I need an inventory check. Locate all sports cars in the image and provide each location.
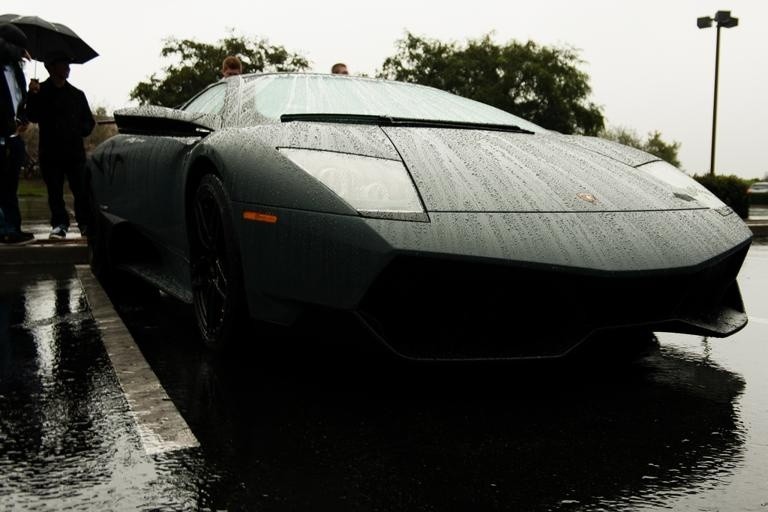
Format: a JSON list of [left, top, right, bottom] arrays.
[[86, 72, 754, 362]]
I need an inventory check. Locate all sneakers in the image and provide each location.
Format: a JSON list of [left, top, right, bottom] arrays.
[[49, 225, 67, 241], [0, 231, 34, 246]]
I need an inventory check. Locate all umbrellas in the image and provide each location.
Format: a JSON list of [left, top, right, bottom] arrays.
[[0, 16, 98, 93]]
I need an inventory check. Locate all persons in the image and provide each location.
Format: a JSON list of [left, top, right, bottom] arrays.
[[331, 63, 348, 74], [221, 55, 242, 80], [18, 54, 96, 240], [0, 23, 35, 243]]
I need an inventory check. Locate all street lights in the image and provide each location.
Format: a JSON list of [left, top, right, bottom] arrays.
[[697, 11, 738, 176]]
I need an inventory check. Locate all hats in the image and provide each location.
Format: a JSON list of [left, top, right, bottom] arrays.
[[1, 23, 32, 61]]
